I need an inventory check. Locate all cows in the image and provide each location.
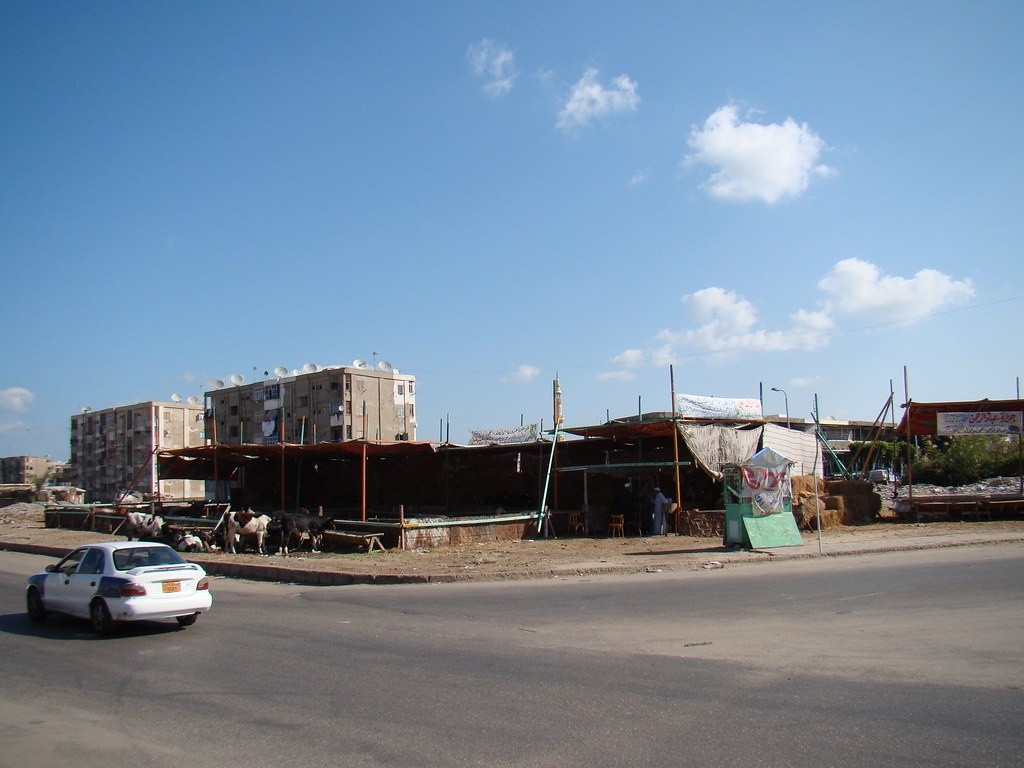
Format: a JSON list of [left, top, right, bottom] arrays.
[[170, 507, 336, 558], [126, 511, 170, 541]]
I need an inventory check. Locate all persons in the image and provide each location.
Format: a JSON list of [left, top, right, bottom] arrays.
[[649, 487, 673, 535]]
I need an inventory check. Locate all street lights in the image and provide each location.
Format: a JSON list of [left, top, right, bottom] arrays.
[[771, 387, 790, 429]]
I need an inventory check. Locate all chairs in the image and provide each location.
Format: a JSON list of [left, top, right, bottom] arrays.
[[147, 551, 163, 565], [566, 509, 585, 538], [85, 551, 98, 568], [607, 513, 625, 539]]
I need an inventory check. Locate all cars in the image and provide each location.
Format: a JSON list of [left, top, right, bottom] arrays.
[[25, 541, 214, 637]]
